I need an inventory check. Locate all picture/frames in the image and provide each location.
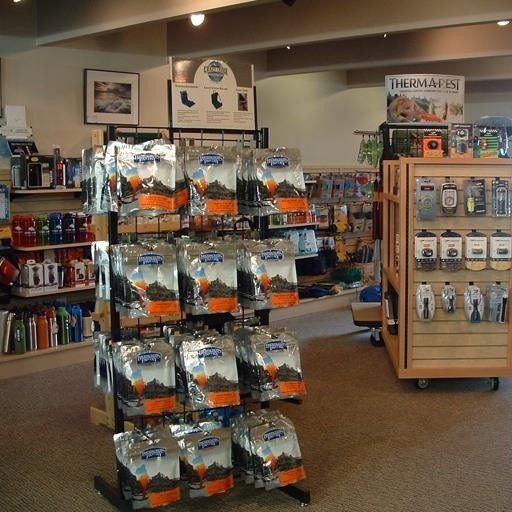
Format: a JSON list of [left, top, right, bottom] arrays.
[[82, 68, 142, 129]]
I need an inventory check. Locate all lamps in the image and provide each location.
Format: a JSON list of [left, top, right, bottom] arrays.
[[189, 13, 208, 28]]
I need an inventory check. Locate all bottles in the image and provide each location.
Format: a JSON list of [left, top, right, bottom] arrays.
[[11, 212, 95, 246], [1, 302, 92, 354]]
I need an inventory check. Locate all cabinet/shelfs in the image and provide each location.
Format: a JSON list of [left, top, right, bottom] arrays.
[[1, 184, 95, 365], [375, 154, 512, 394]]
